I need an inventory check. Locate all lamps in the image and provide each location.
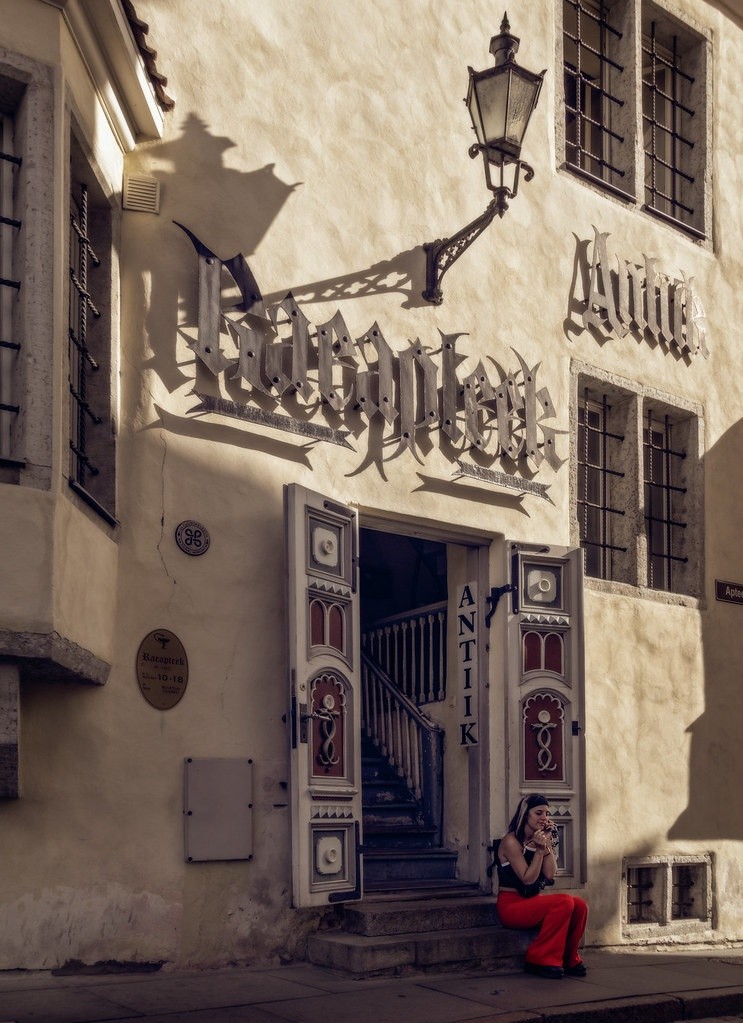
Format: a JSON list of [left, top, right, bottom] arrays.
[[419, 11, 550, 308]]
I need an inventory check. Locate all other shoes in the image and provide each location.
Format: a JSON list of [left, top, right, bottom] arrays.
[[565, 965, 587, 977], [524, 961, 565, 979]]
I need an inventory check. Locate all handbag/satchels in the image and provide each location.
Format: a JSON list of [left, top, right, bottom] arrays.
[[516, 883, 539, 897]]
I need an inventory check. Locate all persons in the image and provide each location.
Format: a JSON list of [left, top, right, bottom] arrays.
[[492, 793, 589, 978]]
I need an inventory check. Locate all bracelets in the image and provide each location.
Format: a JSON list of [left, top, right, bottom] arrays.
[[544, 852, 551, 858]]
